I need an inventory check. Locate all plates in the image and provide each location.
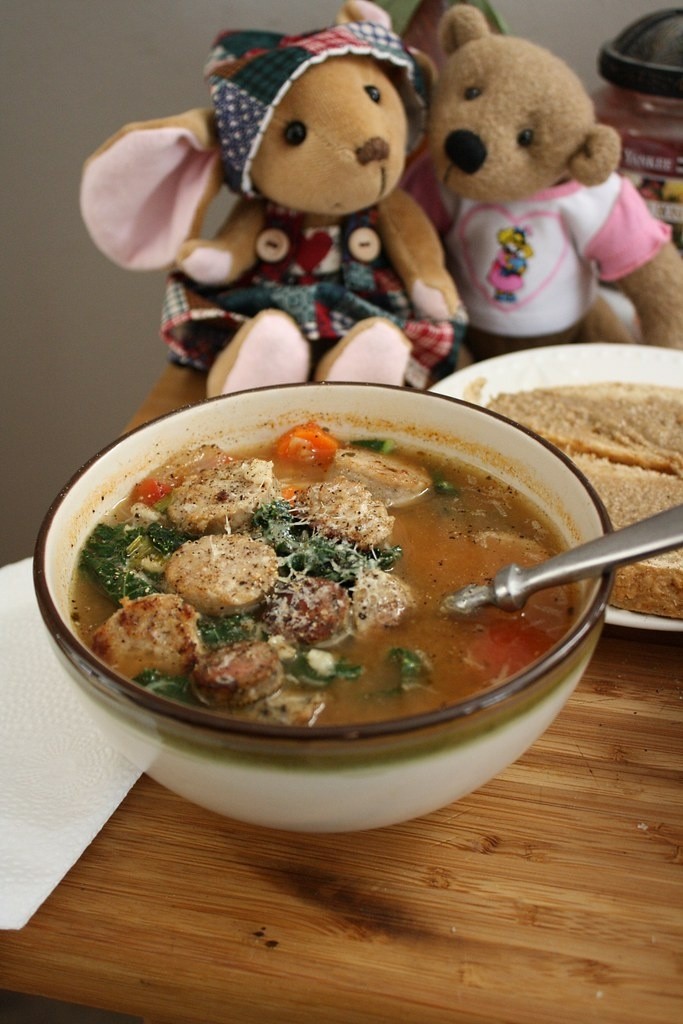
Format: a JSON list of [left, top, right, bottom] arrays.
[[422, 339, 683, 636]]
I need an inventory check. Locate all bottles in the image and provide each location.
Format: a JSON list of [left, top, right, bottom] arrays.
[[580, 8, 683, 255]]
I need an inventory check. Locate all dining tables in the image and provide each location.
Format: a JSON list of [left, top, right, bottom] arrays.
[[0, 359, 683, 1024]]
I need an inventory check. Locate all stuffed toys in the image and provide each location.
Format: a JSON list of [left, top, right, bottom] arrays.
[[393, 0, 681, 359], [73, 3, 468, 392]]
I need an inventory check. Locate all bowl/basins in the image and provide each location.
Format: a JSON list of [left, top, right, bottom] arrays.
[[30, 380, 618, 830]]
